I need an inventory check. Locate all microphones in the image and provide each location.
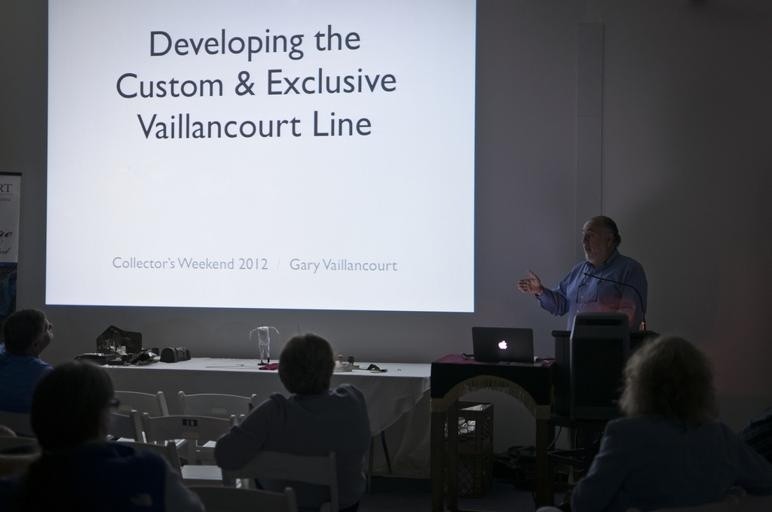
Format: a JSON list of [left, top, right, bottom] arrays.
[[583, 272, 647, 332]]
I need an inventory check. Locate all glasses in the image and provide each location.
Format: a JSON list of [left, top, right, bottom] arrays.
[[95, 397, 122, 415]]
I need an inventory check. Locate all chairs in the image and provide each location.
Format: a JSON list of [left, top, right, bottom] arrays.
[[142, 411, 250, 490], [163, 469, 297, 511], [106, 409, 143, 444], [2, 411, 44, 509], [112, 391, 193, 463], [178, 391, 258, 461]]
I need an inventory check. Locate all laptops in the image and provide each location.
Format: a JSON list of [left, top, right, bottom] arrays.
[[472, 327, 537, 362]]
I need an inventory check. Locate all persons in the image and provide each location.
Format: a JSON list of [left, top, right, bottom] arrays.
[[2, 309, 54, 411], [517, 216, 648, 332], [2, 359, 205, 511], [570, 336, 772, 512], [215, 334, 371, 511]]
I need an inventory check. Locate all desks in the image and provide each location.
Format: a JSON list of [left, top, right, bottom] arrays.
[[80, 356, 430, 481]]
